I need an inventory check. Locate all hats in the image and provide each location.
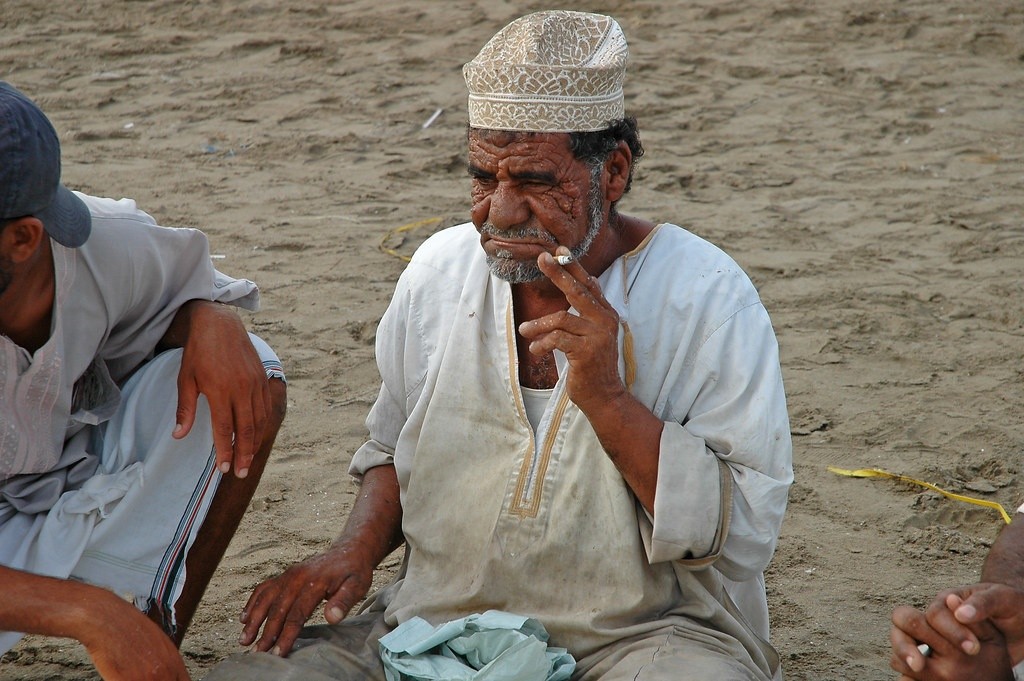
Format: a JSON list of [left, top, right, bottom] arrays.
[[0, 80, 91, 248], [462, 10, 629, 132]]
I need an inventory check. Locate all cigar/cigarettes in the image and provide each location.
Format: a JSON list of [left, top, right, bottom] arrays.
[[553, 256, 572, 265]]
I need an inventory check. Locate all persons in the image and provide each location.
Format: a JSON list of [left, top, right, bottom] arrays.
[[890, 503, 1024, 681], [206, 10, 794, 681], [0, 81, 288, 681]]
